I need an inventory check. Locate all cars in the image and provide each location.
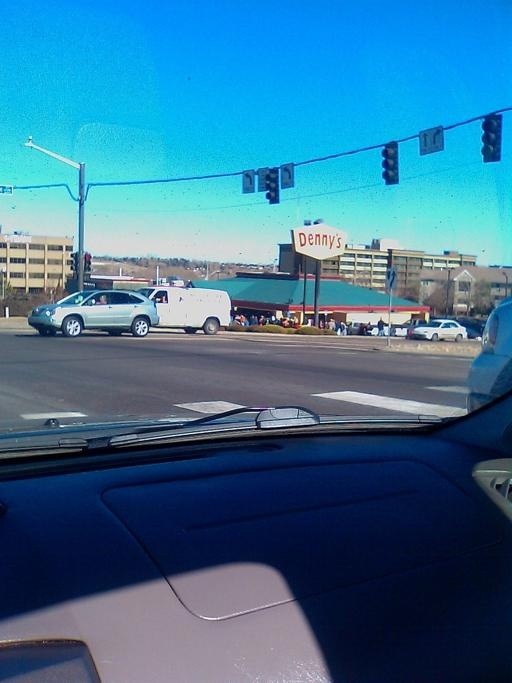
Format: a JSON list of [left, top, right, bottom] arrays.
[[409, 318, 468, 343], [347, 321, 366, 335], [370, 323, 409, 336]]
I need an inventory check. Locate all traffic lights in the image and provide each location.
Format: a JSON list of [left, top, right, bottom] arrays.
[[381, 141, 400, 186], [480, 113, 503, 163], [84, 251, 91, 272], [70, 251, 78, 271], [264, 167, 281, 205]]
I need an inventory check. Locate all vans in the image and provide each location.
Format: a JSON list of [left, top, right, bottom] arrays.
[[134, 286, 232, 335]]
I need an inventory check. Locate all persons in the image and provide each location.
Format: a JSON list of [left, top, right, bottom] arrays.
[[377, 316, 386, 336], [158, 293, 167, 304], [95, 294, 107, 305], [233, 313, 349, 335]]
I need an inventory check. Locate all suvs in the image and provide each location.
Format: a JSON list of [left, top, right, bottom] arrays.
[[26, 288, 161, 338]]
